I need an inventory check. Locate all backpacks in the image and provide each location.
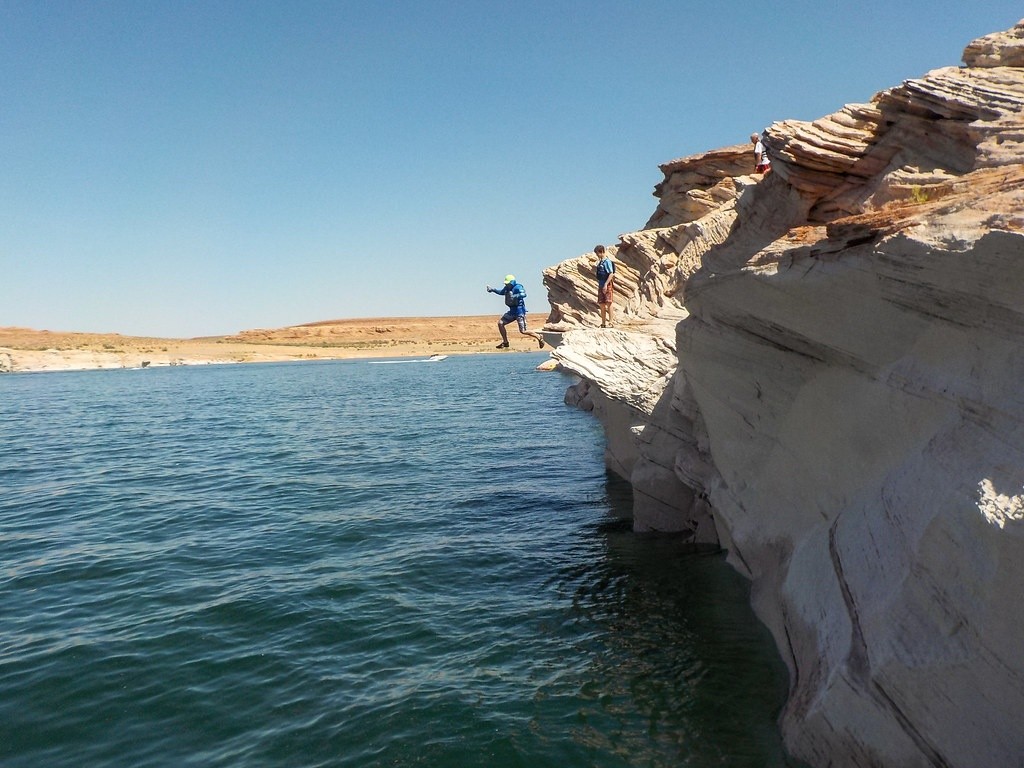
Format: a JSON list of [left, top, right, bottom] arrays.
[[599, 258, 616, 274]]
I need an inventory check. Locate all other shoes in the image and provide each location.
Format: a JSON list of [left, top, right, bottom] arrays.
[[608, 323, 614, 328], [598, 324, 605, 328], [496, 342, 509, 348], [539, 334, 544, 348]]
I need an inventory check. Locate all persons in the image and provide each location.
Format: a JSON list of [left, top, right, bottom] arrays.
[[487, 275, 545, 349], [594, 245, 614, 328]]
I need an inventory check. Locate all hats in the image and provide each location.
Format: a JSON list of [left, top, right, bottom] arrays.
[[503, 275, 516, 286]]
